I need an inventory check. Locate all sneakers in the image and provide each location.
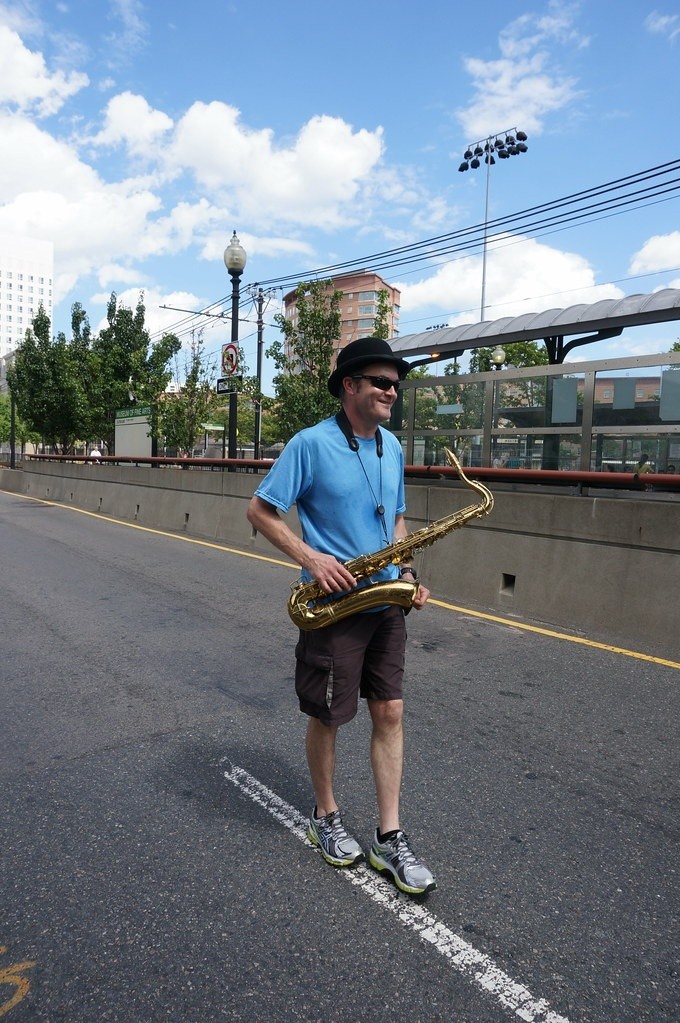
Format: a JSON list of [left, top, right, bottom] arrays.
[[369, 828, 438, 896], [306, 805, 366, 868]]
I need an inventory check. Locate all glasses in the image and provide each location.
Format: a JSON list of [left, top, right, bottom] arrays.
[[351, 375, 400, 393]]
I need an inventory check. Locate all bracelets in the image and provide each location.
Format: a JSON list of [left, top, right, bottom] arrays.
[[401, 568, 416, 580]]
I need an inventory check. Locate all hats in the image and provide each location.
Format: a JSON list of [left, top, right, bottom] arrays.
[[327, 337, 411, 399]]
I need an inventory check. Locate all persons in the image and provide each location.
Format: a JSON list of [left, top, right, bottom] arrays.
[[625, 454, 675, 474], [246, 337, 439, 894], [492, 449, 522, 469], [90, 447, 101, 465], [181, 452, 190, 470]]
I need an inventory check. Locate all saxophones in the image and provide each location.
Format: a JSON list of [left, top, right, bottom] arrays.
[[286, 446, 496, 631]]
